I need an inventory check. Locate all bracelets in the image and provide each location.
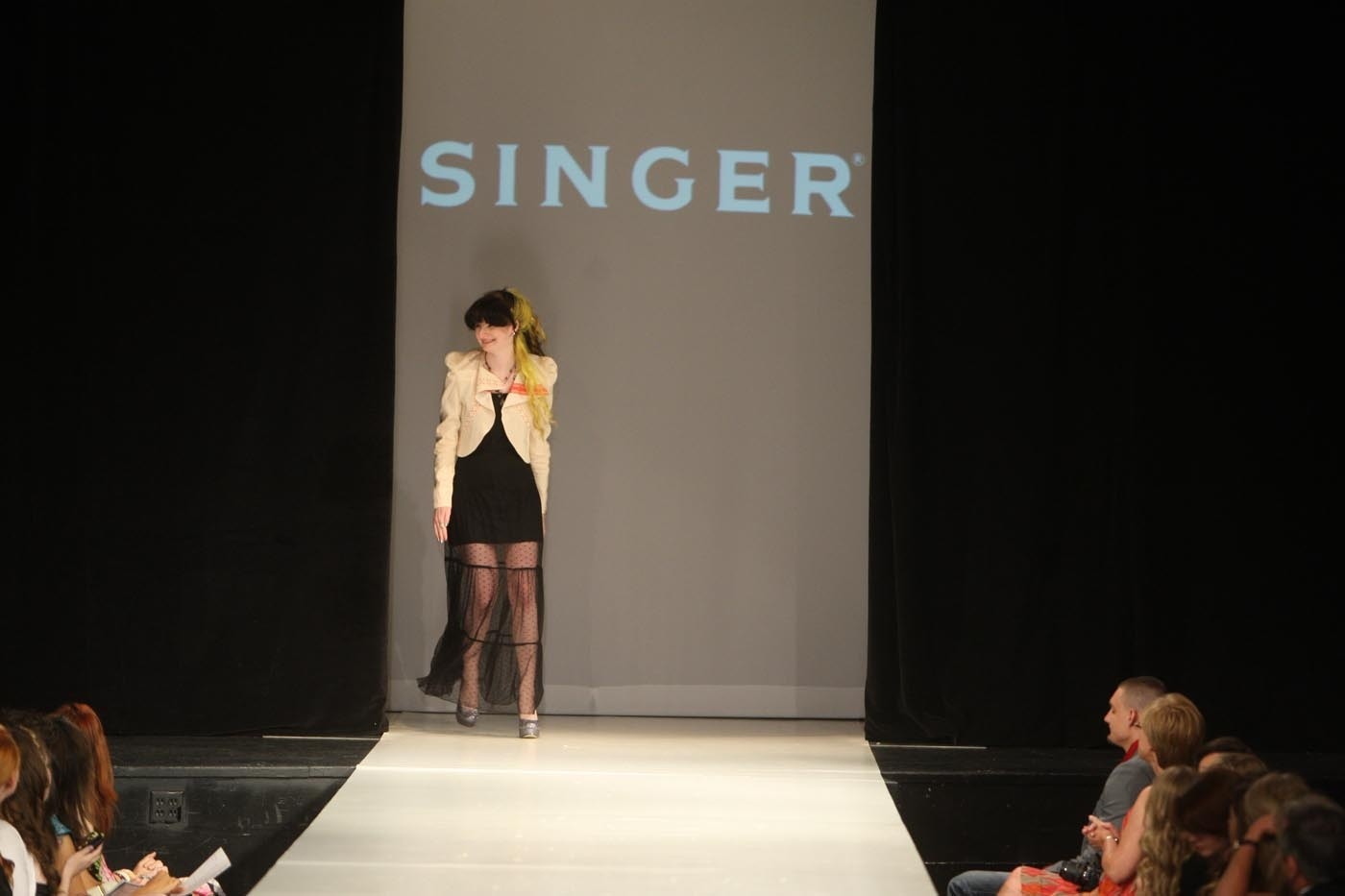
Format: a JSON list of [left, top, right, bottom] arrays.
[[1102, 834, 1121, 844]]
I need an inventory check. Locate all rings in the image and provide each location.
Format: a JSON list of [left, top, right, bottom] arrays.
[[439, 522, 446, 530]]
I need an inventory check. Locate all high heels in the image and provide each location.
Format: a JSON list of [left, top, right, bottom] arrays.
[[518, 712, 540, 740], [456, 698, 479, 727]]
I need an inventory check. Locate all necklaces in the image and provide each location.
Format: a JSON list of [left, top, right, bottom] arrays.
[[484, 350, 519, 399]]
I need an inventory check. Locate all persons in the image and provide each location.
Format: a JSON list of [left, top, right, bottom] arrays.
[[946, 675, 1161, 895], [1132, 730, 1344, 896], [0, 696, 231, 895], [416, 288, 558, 739], [996, 690, 1209, 896]]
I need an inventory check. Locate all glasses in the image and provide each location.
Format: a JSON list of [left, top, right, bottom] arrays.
[[1134, 722, 1144, 732]]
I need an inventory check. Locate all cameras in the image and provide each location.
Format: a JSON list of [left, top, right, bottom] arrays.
[[1058, 858, 1100, 890]]
